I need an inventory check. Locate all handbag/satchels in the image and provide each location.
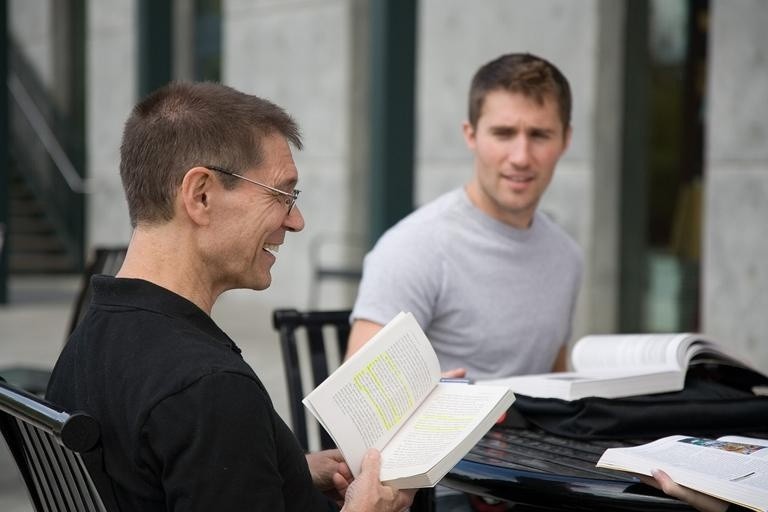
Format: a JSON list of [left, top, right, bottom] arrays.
[[503, 373, 768, 445]]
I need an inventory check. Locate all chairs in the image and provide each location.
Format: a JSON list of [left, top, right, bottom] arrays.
[[270, 306, 359, 460], [0, 374, 119, 509]]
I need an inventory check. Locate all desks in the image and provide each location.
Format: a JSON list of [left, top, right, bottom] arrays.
[[410, 427, 703, 509]]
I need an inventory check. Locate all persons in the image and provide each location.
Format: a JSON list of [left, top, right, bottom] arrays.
[[343, 52, 573, 376], [46, 77, 418, 511], [622, 467, 768, 512]]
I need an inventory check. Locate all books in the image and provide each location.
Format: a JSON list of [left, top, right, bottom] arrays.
[[303, 312, 515, 489], [488, 331, 768, 403], [595, 435, 768, 511]]
